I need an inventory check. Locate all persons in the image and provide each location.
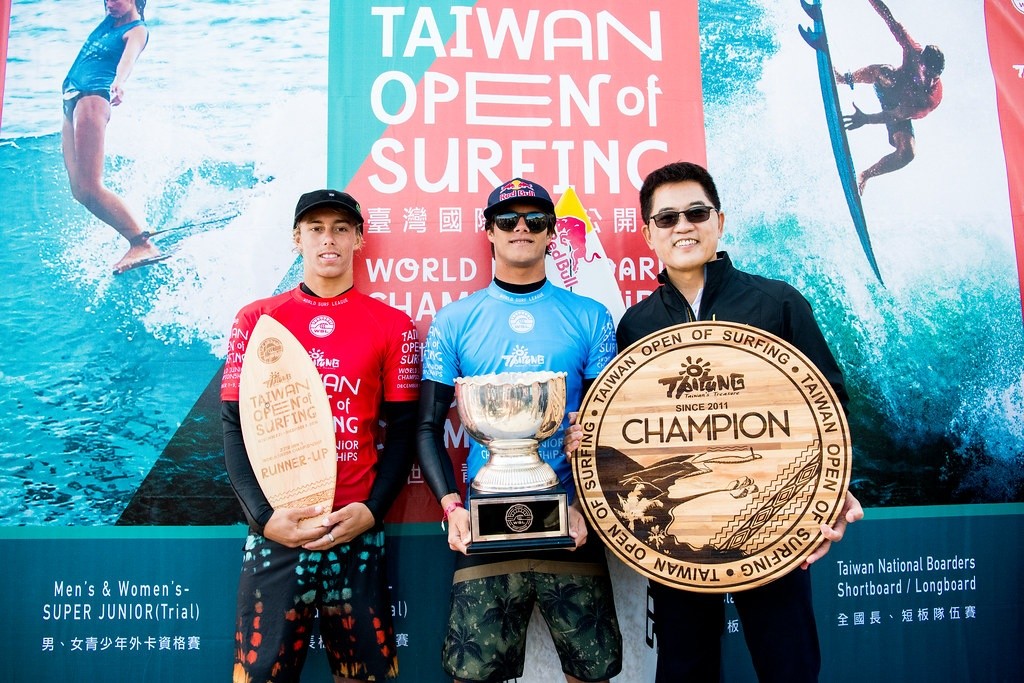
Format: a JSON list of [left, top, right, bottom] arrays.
[[832, 0, 946, 196], [61, 0, 163, 273], [416, 175, 622, 683], [618, 161, 863, 683], [219, 189, 421, 683]]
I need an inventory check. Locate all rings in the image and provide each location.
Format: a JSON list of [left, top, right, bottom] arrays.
[[328, 533, 334, 542]]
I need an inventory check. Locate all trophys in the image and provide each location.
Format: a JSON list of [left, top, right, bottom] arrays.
[[451, 368, 576, 554]]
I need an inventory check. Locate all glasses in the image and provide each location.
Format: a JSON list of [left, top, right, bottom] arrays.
[[647, 206, 718, 228], [489, 211, 555, 233]]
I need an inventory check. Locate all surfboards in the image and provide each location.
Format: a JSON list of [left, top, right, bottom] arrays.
[[111, 249, 178, 274], [795, 0, 887, 291], [235, 313, 338, 530]]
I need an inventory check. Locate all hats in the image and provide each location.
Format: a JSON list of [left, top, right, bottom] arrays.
[[483, 178, 556, 233], [294, 189, 364, 236]]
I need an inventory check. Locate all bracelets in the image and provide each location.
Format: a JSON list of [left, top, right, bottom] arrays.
[[868, 114, 871, 123], [440, 501, 465, 529]]
